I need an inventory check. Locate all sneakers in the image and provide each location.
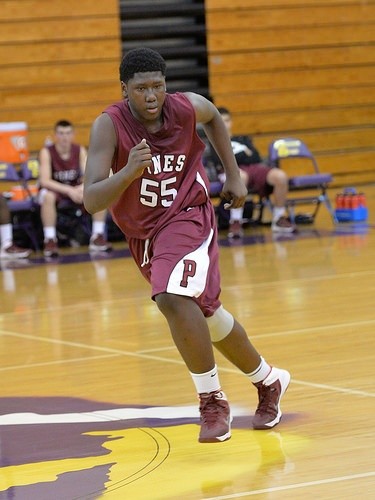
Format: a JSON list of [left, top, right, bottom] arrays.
[[198, 391, 233, 443], [251, 365, 291, 430], [89, 234, 111, 251], [43, 239, 59, 257], [228, 220, 243, 238], [271, 216, 296, 233], [0, 245, 33, 258]]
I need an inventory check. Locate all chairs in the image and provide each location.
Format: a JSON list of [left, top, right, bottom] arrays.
[[8, 139, 338, 252]]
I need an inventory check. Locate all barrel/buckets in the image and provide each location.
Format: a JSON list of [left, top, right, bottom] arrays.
[[0, 122, 28, 164]]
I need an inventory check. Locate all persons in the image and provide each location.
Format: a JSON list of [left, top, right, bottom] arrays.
[[215, 107, 298, 238], [83, 50, 291, 443], [36, 120, 114, 255], [0, 195, 34, 258]]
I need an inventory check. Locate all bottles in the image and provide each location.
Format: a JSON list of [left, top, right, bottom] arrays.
[[336, 191, 367, 210]]
